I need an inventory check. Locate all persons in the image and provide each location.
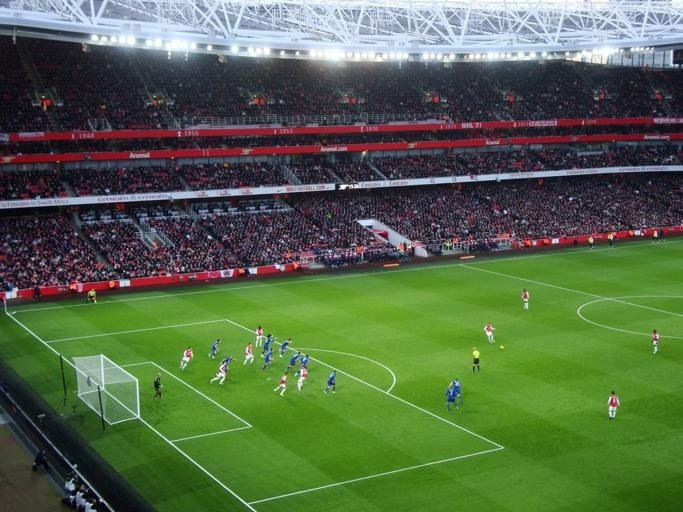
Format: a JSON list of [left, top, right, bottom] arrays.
[[441, 382, 460, 414], [606, 390, 620, 421], [208, 338, 223, 360], [521, 287, 529, 309], [322, 370, 336, 395], [255, 324, 311, 397], [63, 474, 100, 512], [1, 32, 683, 302], [450, 378, 462, 405], [210, 362, 227, 385], [483, 321, 495, 344], [471, 346, 480, 375], [650, 328, 659, 355], [243, 341, 254, 367], [34, 448, 52, 473], [151, 370, 164, 402], [219, 355, 232, 371], [178, 345, 193, 372]]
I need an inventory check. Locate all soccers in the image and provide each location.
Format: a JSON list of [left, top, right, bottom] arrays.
[[500, 346, 504, 350]]
